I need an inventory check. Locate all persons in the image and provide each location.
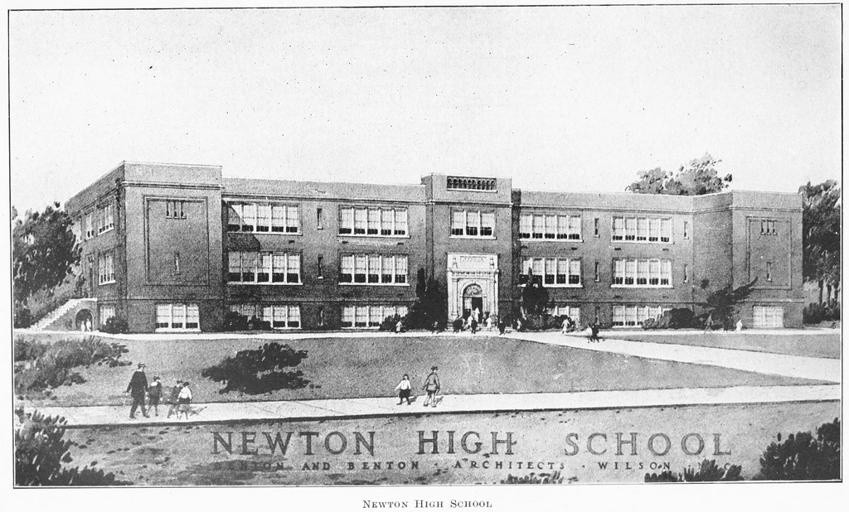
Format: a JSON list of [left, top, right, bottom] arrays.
[[125, 364, 192, 419], [423, 367, 441, 408], [394, 373, 412, 405], [393, 305, 599, 343], [76, 314, 92, 332], [701, 313, 743, 332]]
